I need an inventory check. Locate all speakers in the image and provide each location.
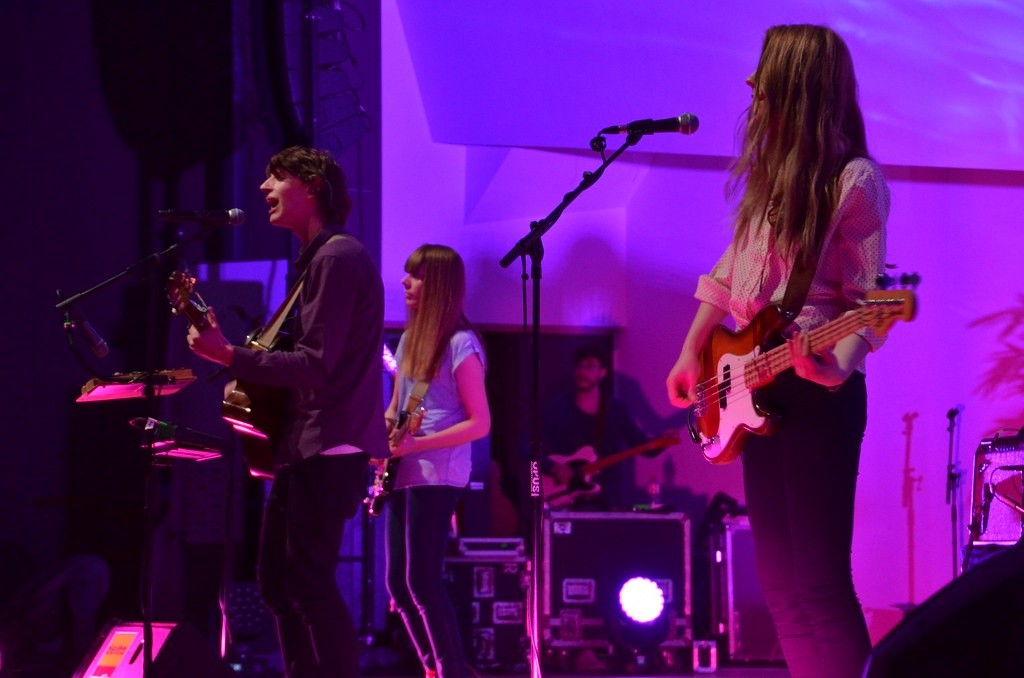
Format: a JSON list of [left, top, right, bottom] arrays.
[[862, 436, 1024, 678], [70, 619, 240, 678], [719, 515, 786, 662]]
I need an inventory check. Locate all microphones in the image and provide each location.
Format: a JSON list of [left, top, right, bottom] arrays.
[[602, 113, 698, 135], [65, 303, 109, 359], [158, 209, 246, 226], [949, 407, 963, 418], [999, 465, 1024, 472]]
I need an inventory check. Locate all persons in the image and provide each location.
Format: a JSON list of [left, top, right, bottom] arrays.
[[666, 23, 892, 678], [186, 145, 492, 678], [535, 341, 680, 674]]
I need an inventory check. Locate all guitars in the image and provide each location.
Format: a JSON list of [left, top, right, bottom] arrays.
[[362, 379, 429, 519], [165, 269, 299, 480], [541, 429, 683, 509], [692, 269, 922, 463]]
